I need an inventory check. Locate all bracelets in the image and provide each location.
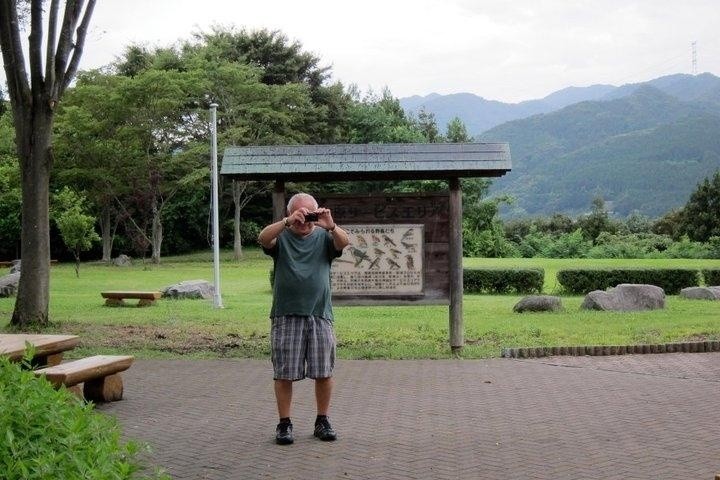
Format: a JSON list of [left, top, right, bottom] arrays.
[[326, 223, 336, 233], [283, 218, 294, 229]]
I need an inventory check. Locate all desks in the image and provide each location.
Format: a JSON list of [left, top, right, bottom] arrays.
[[0, 333, 80, 372]]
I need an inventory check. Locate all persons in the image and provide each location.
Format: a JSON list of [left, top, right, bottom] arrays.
[[256, 192, 350, 446]]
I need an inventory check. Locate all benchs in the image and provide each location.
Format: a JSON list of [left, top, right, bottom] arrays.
[[31, 354, 135, 405], [101, 291, 162, 308]]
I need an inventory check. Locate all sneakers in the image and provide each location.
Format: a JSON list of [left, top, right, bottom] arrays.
[[314, 418, 336, 441], [275, 419, 294, 444]]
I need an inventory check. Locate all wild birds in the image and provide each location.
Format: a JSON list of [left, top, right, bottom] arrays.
[[401, 229, 415, 241], [401, 242, 417, 250], [382, 235, 397, 247], [389, 249, 402, 254], [373, 248, 385, 254], [347, 247, 369, 260], [369, 257, 380, 270], [386, 257, 401, 268], [356, 236, 367, 247], [372, 235, 380, 242], [404, 255, 414, 268]]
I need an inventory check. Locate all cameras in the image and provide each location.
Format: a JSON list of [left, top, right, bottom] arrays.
[[303, 213, 320, 224]]
